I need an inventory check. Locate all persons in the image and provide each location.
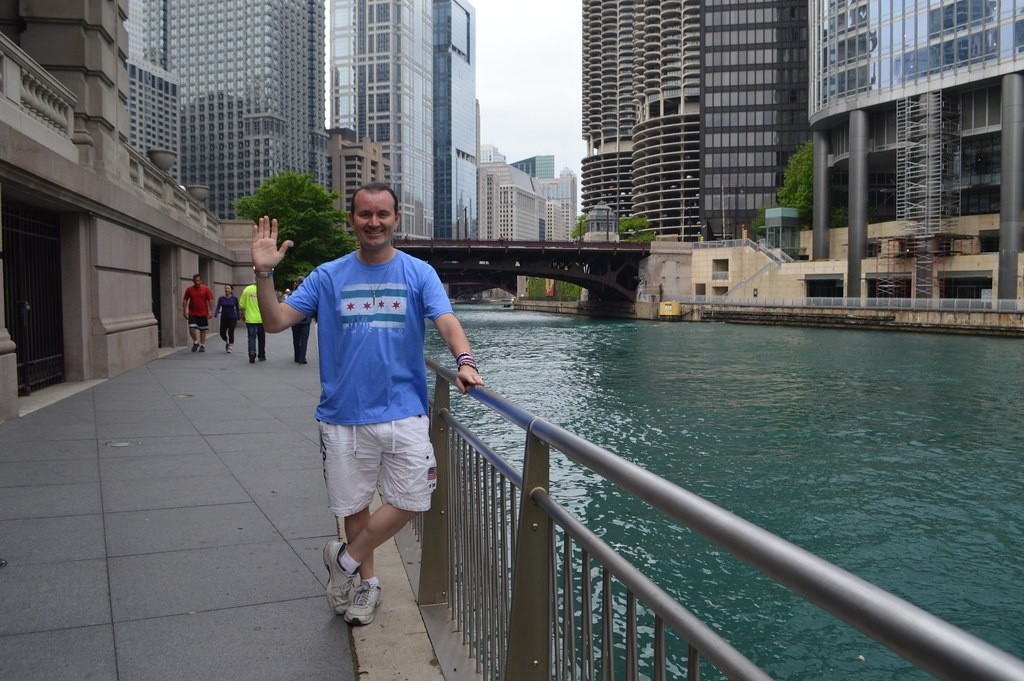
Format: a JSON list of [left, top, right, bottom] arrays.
[[282, 288, 291, 300], [251, 182, 487, 627], [239, 279, 268, 363], [213, 283, 241, 353], [183, 273, 214, 352], [288, 277, 318, 365]]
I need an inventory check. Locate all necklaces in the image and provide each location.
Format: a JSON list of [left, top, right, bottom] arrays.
[[359, 249, 394, 305]]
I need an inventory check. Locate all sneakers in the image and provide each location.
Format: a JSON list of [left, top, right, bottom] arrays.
[[323, 541, 357, 615], [226, 344, 229, 350], [227, 347, 232, 352], [344, 580, 382, 625]]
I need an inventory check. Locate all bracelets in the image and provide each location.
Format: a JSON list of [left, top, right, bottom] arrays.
[[253, 266, 274, 278], [456, 352, 478, 370]]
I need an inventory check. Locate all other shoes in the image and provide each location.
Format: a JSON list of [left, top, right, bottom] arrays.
[[259, 356, 266, 361], [199, 346, 204, 352], [192, 343, 199, 351], [250, 352, 256, 363]]
